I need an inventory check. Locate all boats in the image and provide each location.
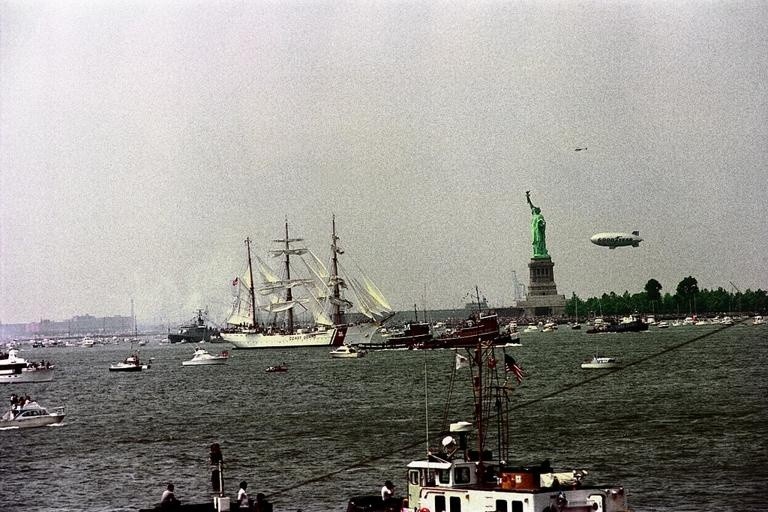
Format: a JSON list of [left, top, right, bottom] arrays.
[[109, 343, 152, 372], [0, 305, 223, 350], [0, 350, 55, 383], [346, 326, 627, 512], [265, 361, 289, 372], [356, 285, 500, 351], [0, 400, 66, 429], [453, 319, 520, 349], [181, 339, 228, 366], [328, 339, 368, 359], [580, 345, 626, 369], [504, 295, 766, 333]]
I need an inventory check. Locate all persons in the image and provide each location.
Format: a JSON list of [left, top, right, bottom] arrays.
[[381, 480, 396, 511], [236, 480, 250, 509], [9, 392, 32, 411], [26, 359, 51, 371], [161, 483, 180, 509], [254, 493, 272, 511], [525, 191, 549, 257]]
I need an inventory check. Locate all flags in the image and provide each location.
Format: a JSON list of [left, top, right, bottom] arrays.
[[505, 355, 524, 384], [454, 353, 467, 371]]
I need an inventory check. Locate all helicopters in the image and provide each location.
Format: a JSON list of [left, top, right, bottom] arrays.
[[572, 145, 588, 152]]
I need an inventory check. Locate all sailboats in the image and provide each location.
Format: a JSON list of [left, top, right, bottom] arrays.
[[220, 212, 396, 350]]
